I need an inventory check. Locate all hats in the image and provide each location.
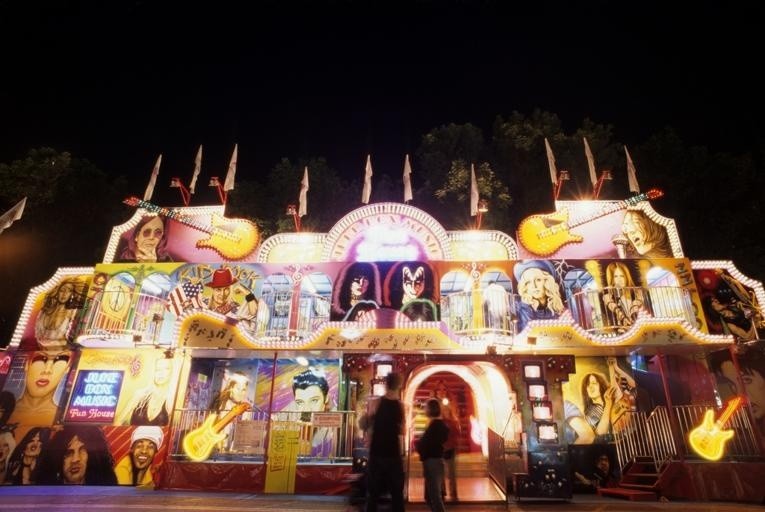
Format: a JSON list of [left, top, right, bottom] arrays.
[[129, 425, 163, 451], [513, 259, 556, 280], [205, 270, 236, 289]]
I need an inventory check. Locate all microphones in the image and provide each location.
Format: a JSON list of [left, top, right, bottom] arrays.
[[612, 234, 629, 259]]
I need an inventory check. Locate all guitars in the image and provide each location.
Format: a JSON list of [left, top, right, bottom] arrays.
[[518, 188, 663, 257], [689, 398, 742, 461], [123, 196, 261, 261], [182, 403, 252, 462]]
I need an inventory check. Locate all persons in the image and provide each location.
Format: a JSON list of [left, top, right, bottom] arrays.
[[562, 400, 596, 447], [602, 260, 647, 334], [417, 398, 448, 511], [571, 452, 613, 495], [290, 370, 338, 461], [358, 371, 408, 512], [386, 262, 435, 326], [514, 267, 566, 334], [440, 401, 462, 498], [622, 207, 673, 259], [332, 260, 383, 322], [481, 279, 511, 331], [584, 355, 639, 438]]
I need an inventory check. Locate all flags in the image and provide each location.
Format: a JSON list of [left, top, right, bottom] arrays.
[[403, 155, 414, 203], [470, 165, 480, 215], [0, 197, 26, 233], [224, 143, 239, 190], [623, 146, 640, 194], [545, 139, 557, 184], [298, 165, 310, 217], [584, 139, 598, 187], [143, 155, 163, 203], [189, 144, 202, 196], [361, 154, 373, 205]]
